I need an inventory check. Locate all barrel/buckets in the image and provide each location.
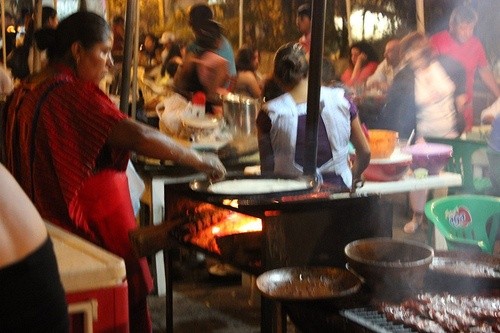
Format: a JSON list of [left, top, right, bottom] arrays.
[[218, 93, 263, 144]]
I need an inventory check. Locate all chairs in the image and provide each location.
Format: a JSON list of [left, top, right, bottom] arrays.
[[424, 135, 493, 195], [425, 194, 500, 255]]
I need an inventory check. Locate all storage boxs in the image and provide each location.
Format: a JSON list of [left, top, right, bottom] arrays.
[[45, 222, 130, 333]]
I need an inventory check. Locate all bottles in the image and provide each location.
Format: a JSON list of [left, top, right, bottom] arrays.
[[185, 91, 207, 121]]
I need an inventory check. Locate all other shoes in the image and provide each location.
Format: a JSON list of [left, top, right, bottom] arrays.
[[404, 221, 423, 233]]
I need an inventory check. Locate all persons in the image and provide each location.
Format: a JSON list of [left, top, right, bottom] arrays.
[[0, 0, 500, 194], [0, 10, 227, 333], [0, 162, 69, 333]]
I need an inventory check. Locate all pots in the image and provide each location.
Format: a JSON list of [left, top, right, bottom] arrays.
[[186, 165, 323, 204]]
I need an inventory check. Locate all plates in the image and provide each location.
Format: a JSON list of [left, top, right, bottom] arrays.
[[429, 251, 500, 291], [257, 265, 361, 303]]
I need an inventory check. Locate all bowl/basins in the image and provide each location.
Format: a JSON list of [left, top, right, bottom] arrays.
[[344, 238, 436, 303], [350, 152, 413, 183], [363, 128, 399, 160], [405, 143, 454, 175]]
